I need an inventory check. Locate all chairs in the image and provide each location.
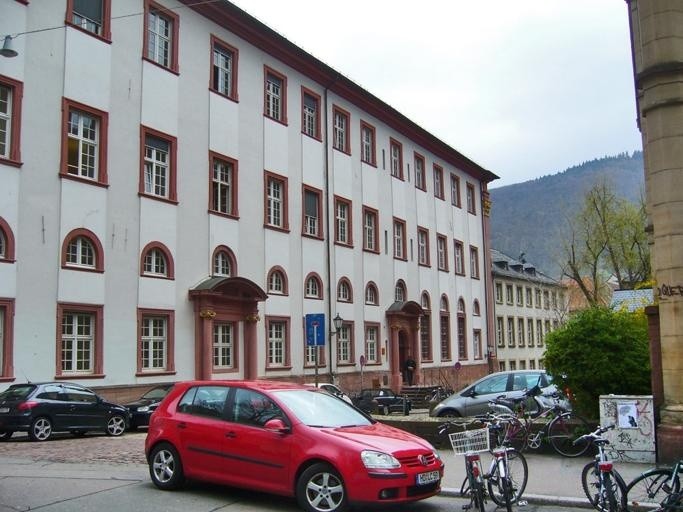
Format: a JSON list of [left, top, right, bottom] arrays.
[[236, 391, 256, 421]]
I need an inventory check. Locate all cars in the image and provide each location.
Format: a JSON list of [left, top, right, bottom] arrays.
[[431, 369, 577, 417], [303, 381, 355, 405], [143, 379, 445, 512], [0, 381, 131, 443], [122, 381, 221, 432], [351, 387, 412, 416]]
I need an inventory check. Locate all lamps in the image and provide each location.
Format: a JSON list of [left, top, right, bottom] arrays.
[[329, 312, 343, 336]]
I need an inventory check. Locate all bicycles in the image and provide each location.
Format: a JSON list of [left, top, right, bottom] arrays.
[[437, 376, 683, 512]]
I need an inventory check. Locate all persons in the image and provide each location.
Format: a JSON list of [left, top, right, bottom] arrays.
[[406, 356, 416, 386]]
